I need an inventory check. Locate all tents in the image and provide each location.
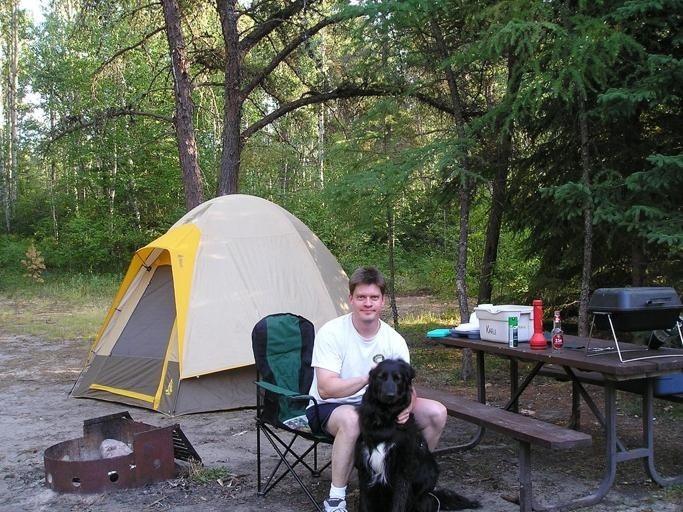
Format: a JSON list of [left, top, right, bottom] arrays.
[[68, 194, 352, 417]]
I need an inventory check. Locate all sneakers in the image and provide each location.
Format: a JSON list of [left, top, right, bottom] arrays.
[[323, 497, 348, 512]]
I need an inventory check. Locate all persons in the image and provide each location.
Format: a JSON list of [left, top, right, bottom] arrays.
[[305, 267, 447, 512]]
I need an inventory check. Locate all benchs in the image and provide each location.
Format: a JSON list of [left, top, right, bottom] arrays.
[[538, 364, 683, 432], [414, 384, 593, 512]]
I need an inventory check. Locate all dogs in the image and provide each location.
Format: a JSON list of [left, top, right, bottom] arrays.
[[354, 357, 484, 512]]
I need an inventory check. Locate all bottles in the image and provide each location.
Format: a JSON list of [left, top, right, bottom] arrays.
[[551, 311, 565, 351], [508, 316, 518, 349]]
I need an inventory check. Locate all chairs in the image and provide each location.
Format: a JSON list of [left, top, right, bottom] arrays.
[[252, 313, 333, 512]]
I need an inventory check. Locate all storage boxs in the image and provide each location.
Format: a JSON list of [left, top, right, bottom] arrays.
[[584, 287, 683, 363], [624, 372, 683, 400], [474, 305, 534, 344]]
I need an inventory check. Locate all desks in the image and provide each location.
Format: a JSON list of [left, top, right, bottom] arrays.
[[423, 333, 683, 512]]
